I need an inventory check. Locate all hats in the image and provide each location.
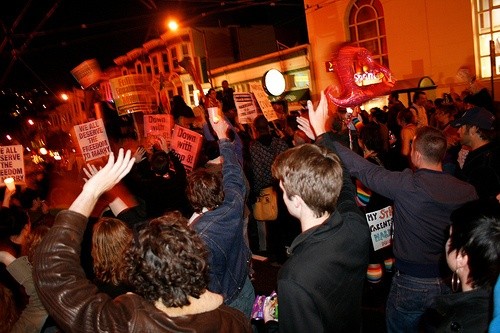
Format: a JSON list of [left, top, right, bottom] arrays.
[[463, 94, 483, 107], [450, 107, 495, 130]]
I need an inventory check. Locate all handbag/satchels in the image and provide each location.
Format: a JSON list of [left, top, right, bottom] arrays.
[[252, 186, 278, 220]]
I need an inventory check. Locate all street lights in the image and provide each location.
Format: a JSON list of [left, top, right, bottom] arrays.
[[167, 19, 213, 89]]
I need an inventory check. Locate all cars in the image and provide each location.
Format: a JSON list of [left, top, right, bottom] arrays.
[[0, 132, 46, 202], [42, 129, 77, 177]]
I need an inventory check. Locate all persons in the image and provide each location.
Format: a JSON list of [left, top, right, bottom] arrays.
[[271, 90, 370, 333], [0, 81, 500, 333]]
